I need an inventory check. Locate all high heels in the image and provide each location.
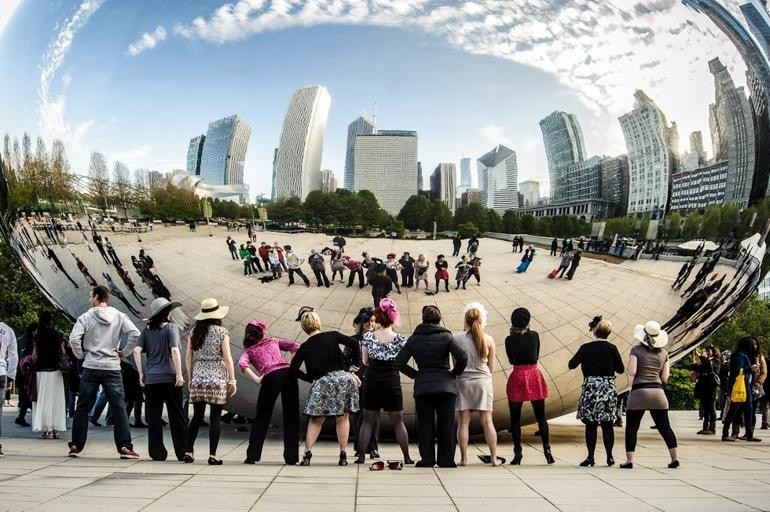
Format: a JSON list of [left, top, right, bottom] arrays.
[[607, 457, 614, 466], [579, 458, 595, 467], [544, 449, 555, 464], [39, 431, 53, 439], [53, 431, 60, 439], [338, 451, 348, 466], [510, 454, 523, 465], [300, 450, 312, 466]]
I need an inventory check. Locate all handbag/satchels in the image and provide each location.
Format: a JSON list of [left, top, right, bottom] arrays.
[[709, 357, 721, 388], [58, 353, 74, 374], [731, 368, 747, 403]]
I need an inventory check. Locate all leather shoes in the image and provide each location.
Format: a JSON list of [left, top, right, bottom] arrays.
[[15, 417, 30, 426], [386, 461, 404, 470], [184, 454, 194, 463], [370, 450, 380, 459], [620, 463, 632, 468], [369, 460, 389, 471], [88, 414, 209, 427], [477, 455, 506, 463], [209, 458, 222, 465], [697, 430, 761, 441], [668, 461, 679, 468]]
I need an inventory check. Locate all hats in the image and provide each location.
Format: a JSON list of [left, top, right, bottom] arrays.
[[310, 249, 321, 255], [142, 297, 183, 322], [354, 307, 374, 324], [333, 248, 342, 252], [379, 297, 399, 321], [197, 298, 230, 321], [511, 308, 530, 328], [248, 320, 268, 330], [589, 315, 602, 331], [463, 301, 488, 327], [634, 320, 669, 348]]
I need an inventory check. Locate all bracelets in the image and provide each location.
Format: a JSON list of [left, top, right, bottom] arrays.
[[228, 379, 237, 384], [188, 376, 191, 381]]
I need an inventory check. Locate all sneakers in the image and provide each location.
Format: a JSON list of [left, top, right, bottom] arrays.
[[120, 447, 140, 459], [69, 445, 80, 457]]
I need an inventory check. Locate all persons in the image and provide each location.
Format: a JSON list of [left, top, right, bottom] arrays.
[[237, 318, 302, 464], [392, 304, 469, 467], [355, 298, 414, 465], [0, 306, 248, 439], [618, 320, 680, 468], [225, 222, 482, 307], [132, 296, 191, 460], [504, 307, 555, 465], [687, 337, 770, 442], [343, 306, 383, 459], [452, 301, 504, 467], [640, 224, 760, 335], [565, 315, 625, 466], [290, 305, 363, 466], [550, 236, 627, 280], [11, 214, 197, 313], [512, 235, 535, 273], [183, 297, 237, 465], [67, 285, 143, 459]]
